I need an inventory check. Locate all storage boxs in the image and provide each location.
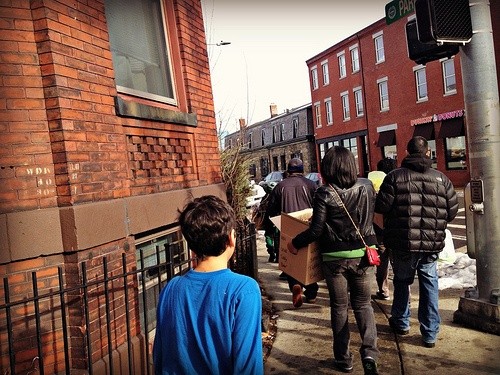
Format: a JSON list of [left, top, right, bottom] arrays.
[[268, 208, 325, 285]]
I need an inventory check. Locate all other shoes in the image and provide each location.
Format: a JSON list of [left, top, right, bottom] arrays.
[[423, 339, 436, 348], [309, 297, 317, 303], [389, 316, 409, 337], [341, 364, 354, 373], [376, 290, 390, 300], [362, 356, 380, 375], [292, 284, 304, 308]]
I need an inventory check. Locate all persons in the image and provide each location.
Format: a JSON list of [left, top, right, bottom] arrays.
[[152, 195, 264, 375], [286, 146, 378, 375], [253, 156, 413, 310], [375, 136, 459, 348]]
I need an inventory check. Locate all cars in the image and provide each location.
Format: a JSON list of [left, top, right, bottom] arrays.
[[244, 180, 267, 209], [258, 172, 286, 194], [305, 173, 322, 186]]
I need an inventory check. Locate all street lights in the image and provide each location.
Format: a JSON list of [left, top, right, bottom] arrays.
[[207, 40, 232, 46]]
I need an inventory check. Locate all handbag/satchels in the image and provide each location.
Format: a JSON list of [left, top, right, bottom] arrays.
[[366, 245, 381, 266]]
